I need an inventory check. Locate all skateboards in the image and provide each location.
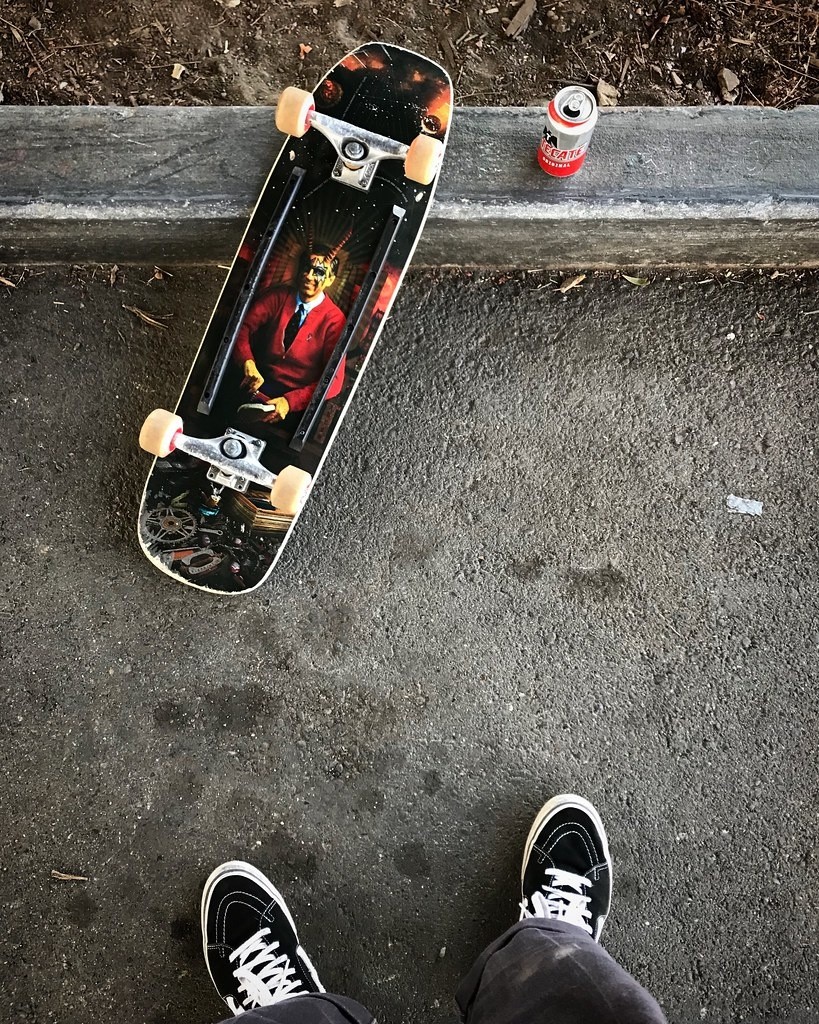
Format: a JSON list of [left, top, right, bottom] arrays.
[[136, 38, 456, 600]]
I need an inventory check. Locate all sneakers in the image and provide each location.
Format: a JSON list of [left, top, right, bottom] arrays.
[[200, 860, 326, 1017], [519, 793, 612, 945]]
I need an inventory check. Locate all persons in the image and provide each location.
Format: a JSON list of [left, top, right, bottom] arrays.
[[234, 244, 347, 423], [200, 794, 668, 1024]]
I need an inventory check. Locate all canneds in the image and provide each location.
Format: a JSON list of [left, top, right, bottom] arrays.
[[537, 85, 598, 177]]
